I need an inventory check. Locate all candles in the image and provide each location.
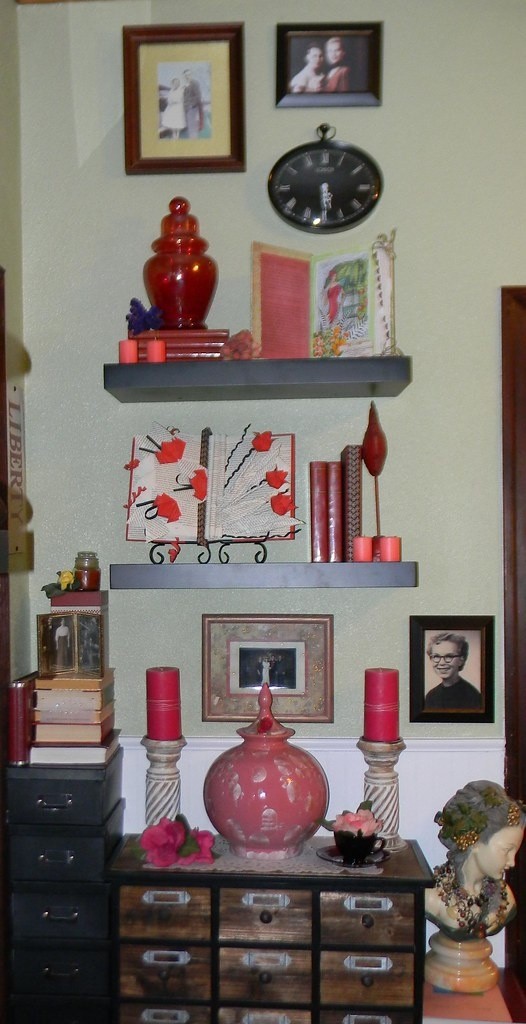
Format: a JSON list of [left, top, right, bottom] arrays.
[[145, 665, 180, 736], [363, 667, 401, 742]]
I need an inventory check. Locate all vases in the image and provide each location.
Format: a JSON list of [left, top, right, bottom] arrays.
[[334, 829, 386, 862]]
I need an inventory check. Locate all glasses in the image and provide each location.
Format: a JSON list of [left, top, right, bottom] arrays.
[[432, 654, 461, 663]]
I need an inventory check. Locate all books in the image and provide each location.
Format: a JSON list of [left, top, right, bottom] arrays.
[[29, 667, 121, 768], [309, 443, 362, 562]]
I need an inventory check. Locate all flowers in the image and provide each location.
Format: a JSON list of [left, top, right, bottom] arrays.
[[129, 812, 218, 866], [314, 800, 388, 835], [42, 569, 78, 597]]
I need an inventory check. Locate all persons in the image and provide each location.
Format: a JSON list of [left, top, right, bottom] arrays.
[[42, 619, 94, 669], [256, 654, 290, 688], [290, 37, 349, 93], [423, 779, 526, 942], [425, 632, 481, 708], [164, 70, 206, 136]]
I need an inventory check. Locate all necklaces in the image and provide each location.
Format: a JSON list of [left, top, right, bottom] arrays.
[[428, 862, 508, 937]]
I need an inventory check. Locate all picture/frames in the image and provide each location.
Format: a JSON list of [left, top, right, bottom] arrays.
[[407, 614, 494, 724], [199, 611, 336, 724], [273, 19, 382, 107], [120, 22, 245, 176]]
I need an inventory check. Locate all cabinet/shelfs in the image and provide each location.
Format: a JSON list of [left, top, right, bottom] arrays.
[[0, 750, 120, 1024], [107, 834, 437, 1024], [104, 352, 423, 590]]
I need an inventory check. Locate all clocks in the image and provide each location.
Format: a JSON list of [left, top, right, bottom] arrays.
[[266, 128, 384, 232]]
